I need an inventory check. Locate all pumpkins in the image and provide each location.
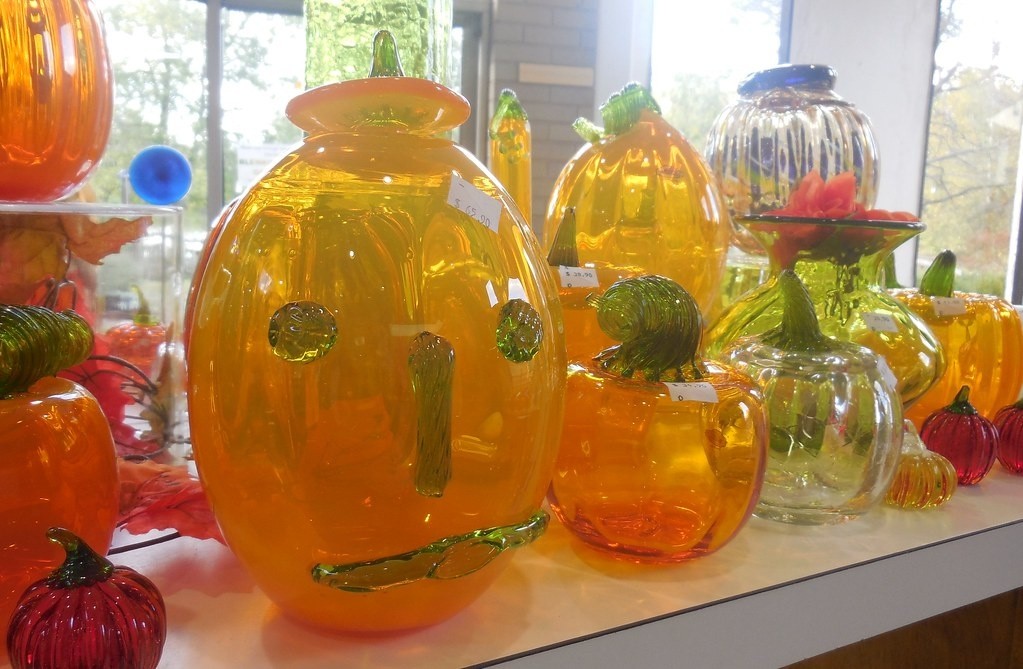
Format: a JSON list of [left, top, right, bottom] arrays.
[[7, 250, 1022, 669]]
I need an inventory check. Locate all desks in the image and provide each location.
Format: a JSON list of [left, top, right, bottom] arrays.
[[103, 462, 1023, 669]]
